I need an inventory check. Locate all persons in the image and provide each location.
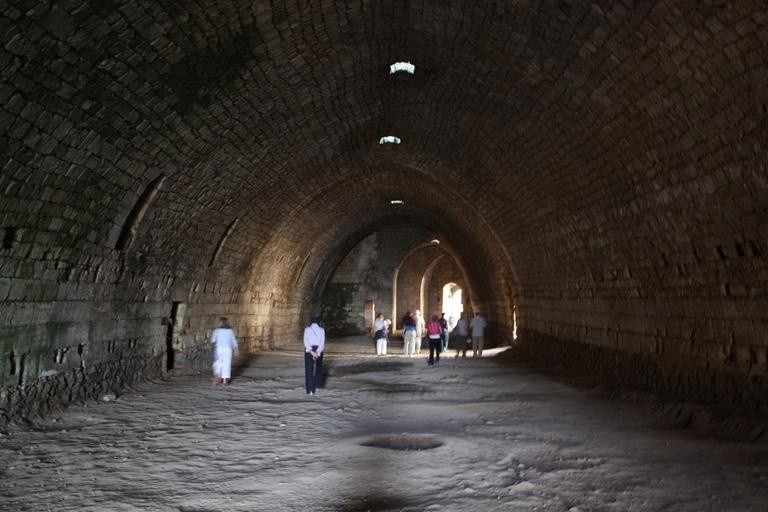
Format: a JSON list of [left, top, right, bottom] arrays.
[[410, 309, 426, 355], [303, 314, 326, 395], [426, 313, 443, 366], [399, 311, 417, 356], [453, 311, 470, 359], [371, 313, 391, 356], [468, 312, 488, 358], [438, 312, 448, 354], [210, 316, 240, 386]]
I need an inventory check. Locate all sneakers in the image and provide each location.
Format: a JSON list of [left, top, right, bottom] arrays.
[[212, 378, 229, 388], [309, 388, 321, 395], [377, 346, 483, 369]]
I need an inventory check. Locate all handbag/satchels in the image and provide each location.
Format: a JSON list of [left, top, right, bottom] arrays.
[[209, 343, 218, 362]]
